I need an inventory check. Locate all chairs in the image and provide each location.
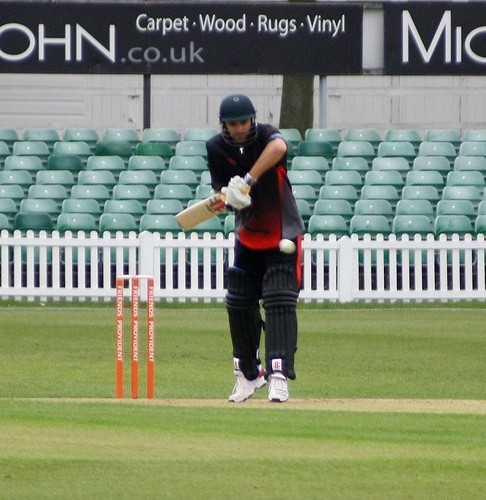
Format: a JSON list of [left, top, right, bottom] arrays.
[[0, 128, 486, 268]]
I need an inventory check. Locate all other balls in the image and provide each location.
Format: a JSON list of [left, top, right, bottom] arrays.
[[278, 238, 295, 253]]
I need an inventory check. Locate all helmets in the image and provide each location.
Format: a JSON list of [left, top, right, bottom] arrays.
[[219, 94, 256, 121]]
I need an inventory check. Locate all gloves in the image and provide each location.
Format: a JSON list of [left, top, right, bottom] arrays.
[[221, 172, 257, 211]]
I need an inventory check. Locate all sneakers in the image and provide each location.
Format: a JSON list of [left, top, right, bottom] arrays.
[[228, 357, 267, 403], [268, 358, 289, 401]]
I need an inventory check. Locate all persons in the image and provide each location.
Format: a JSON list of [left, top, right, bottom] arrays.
[[205, 93, 302, 402]]
